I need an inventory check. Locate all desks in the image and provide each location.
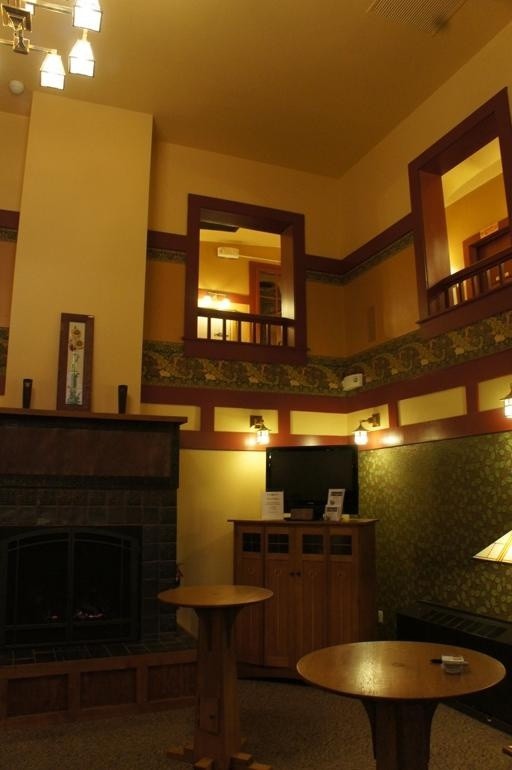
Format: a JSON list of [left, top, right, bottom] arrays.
[[297, 641, 506, 769], [156, 585, 274, 769]]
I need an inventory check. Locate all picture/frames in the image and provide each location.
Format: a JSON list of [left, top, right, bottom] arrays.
[[56, 312, 94, 410]]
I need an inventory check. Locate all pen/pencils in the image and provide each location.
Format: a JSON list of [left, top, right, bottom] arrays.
[[431, 659, 469, 665]]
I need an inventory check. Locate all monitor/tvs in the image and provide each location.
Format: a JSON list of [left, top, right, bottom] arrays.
[[266, 444, 359, 521]]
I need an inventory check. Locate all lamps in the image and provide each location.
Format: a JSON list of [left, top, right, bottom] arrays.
[[353, 413, 381, 445], [1, 0, 102, 91], [500, 383, 512, 417], [249, 415, 271, 444]]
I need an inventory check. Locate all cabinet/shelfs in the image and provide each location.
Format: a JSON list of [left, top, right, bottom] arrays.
[[227, 512, 380, 679]]
